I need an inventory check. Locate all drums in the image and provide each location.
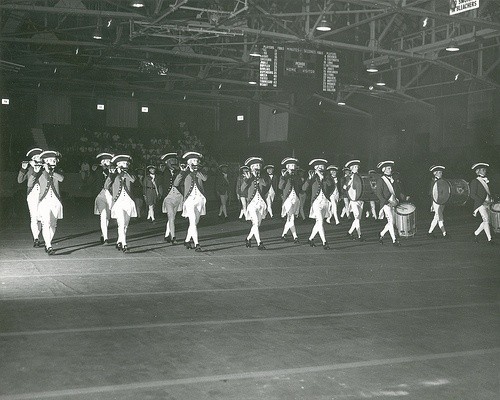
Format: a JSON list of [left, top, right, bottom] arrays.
[[393, 202, 417, 238], [431, 177, 471, 207], [488, 200, 500, 234], [345, 172, 380, 202]]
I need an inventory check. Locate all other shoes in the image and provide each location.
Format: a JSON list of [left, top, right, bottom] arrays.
[[239, 216, 497, 250], [45, 246, 55, 255], [100, 237, 108, 245], [116, 242, 128, 253], [33, 237, 40, 248], [146, 216, 201, 252]]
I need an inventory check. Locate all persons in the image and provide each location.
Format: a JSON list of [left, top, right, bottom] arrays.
[[18, 148, 63, 254], [78, 134, 201, 172], [160, 151, 207, 251], [427, 166, 450, 239], [469, 163, 498, 246], [132, 165, 159, 223], [341, 160, 401, 247], [216, 164, 230, 218], [93, 152, 137, 253], [236, 156, 306, 249], [301, 159, 335, 249], [326, 165, 341, 226]]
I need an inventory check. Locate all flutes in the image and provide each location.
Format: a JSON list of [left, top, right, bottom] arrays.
[[33, 164, 56, 167], [108, 166, 132, 170], [91, 164, 114, 166], [177, 163, 205, 168], [16, 159, 42, 163]]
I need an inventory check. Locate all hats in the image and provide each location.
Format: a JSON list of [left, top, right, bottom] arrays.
[[96, 153, 114, 162], [147, 166, 157, 170], [327, 166, 338, 171], [219, 164, 229, 168], [472, 163, 489, 170], [309, 158, 328, 166], [245, 157, 264, 166], [26, 148, 44, 158], [161, 152, 178, 161], [344, 160, 360, 168], [368, 161, 394, 174], [182, 152, 204, 164], [281, 158, 305, 173], [240, 165, 250, 171], [111, 155, 133, 166], [429, 164, 446, 171], [40, 151, 58, 159], [264, 165, 275, 170]]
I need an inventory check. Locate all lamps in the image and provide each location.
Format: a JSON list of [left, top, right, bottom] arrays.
[[92, 0, 464, 86]]
